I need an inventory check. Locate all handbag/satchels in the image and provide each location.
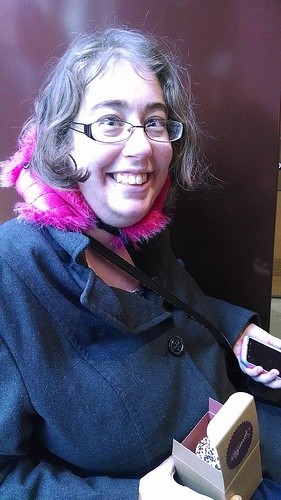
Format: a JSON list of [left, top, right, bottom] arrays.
[[225, 350, 281, 407]]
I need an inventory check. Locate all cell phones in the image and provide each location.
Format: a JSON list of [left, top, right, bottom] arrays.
[[241, 335, 281, 380]]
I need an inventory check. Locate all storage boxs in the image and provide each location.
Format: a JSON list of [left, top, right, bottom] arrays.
[[171, 392, 264, 500]]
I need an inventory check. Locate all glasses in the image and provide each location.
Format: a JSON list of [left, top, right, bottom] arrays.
[[69, 116, 184, 144]]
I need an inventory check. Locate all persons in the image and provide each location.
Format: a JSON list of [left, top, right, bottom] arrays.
[[1, 27, 280, 500]]
